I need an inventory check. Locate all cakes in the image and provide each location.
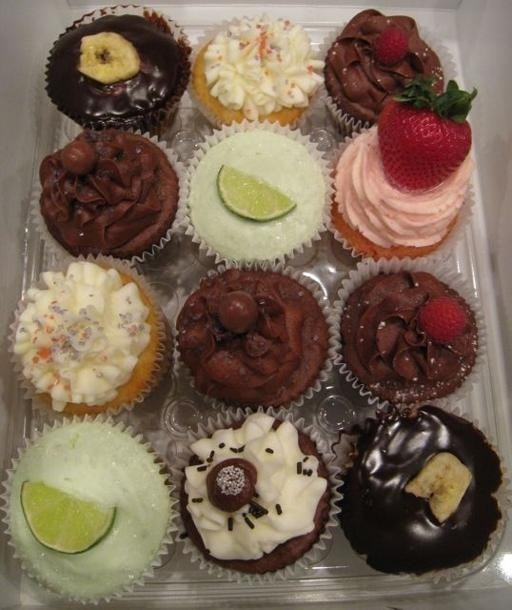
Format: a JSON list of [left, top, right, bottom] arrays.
[[42, 5, 192, 135], [187, 13, 323, 128], [176, 266, 327, 409], [328, 77, 476, 268], [178, 412, 332, 575], [9, 421, 172, 603], [35, 127, 179, 258], [321, 6, 457, 137], [339, 406, 504, 575], [16, 259, 162, 416], [182, 117, 332, 268], [342, 271, 480, 402]]
